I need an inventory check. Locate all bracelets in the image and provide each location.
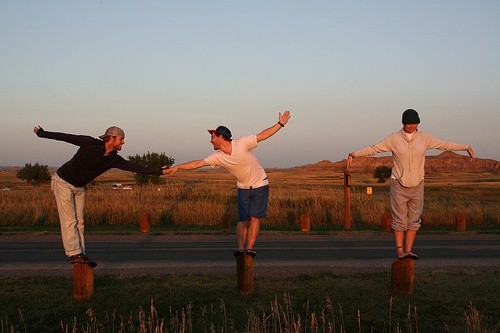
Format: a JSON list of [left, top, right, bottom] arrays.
[[278, 122, 284, 127], [349, 153, 354, 158]]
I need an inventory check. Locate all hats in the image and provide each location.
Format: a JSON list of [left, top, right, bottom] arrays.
[[98, 126, 124, 139], [208, 126, 232, 139], [402, 109, 420, 124]]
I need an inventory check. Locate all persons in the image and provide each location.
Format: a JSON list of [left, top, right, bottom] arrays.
[[346, 109, 475, 258], [33, 125, 168, 267], [162, 110, 291, 257]]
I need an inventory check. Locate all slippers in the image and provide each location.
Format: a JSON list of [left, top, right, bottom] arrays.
[[234, 249, 245, 256], [245, 248, 256, 257]]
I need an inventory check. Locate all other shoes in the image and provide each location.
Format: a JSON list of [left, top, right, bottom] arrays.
[[407, 252, 419, 261], [399, 253, 408, 259], [70, 253, 97, 268]]
